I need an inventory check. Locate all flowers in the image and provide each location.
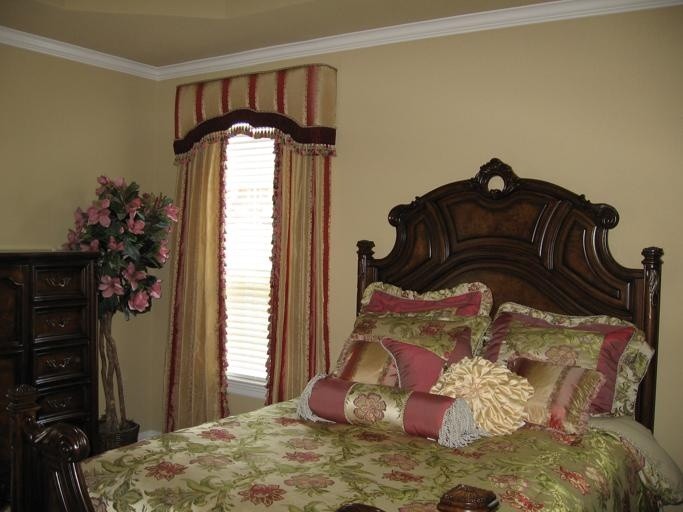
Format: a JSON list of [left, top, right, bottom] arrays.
[[59, 174, 176, 421]]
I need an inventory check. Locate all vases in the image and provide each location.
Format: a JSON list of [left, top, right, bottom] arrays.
[[89, 418, 136, 453]]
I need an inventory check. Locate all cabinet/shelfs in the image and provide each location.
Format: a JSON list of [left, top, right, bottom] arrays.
[[0, 251, 97, 461]]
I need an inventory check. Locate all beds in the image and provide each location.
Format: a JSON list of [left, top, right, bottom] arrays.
[[5, 158, 683, 509]]
